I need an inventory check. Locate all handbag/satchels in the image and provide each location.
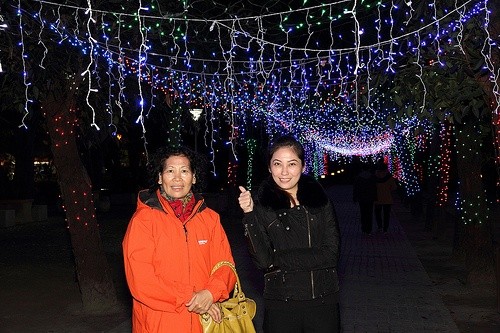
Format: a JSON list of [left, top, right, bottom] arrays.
[[198, 261, 256, 333]]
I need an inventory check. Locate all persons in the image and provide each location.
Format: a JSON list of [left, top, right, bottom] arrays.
[[238, 136, 342, 332], [121, 146, 236, 332], [353, 166, 378, 234], [375, 162, 396, 233]]
